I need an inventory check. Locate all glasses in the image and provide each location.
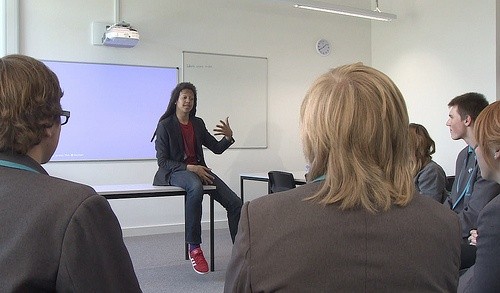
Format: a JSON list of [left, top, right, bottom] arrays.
[[59, 110, 70, 124]]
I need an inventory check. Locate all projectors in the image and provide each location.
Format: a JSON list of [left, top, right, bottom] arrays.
[[102, 24, 139, 49]]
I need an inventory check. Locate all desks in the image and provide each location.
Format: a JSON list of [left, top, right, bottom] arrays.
[[240, 173, 306, 205], [88, 184, 219, 272]]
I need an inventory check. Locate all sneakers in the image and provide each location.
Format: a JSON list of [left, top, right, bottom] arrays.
[[189, 247, 210, 274]]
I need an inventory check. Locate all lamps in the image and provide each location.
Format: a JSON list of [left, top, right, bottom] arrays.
[[292, 0, 397, 24]]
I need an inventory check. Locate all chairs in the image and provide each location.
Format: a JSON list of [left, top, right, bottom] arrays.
[[267, 170, 296, 196]]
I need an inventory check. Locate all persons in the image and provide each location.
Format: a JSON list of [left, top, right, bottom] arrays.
[[406, 123, 449, 201], [225, 65, 459, 293], [443, 92, 499, 268], [0, 54, 142, 293], [456, 100, 500, 293], [150, 83, 242, 275]]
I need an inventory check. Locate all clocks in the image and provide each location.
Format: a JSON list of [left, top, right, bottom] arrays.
[[314, 37, 332, 56]]
